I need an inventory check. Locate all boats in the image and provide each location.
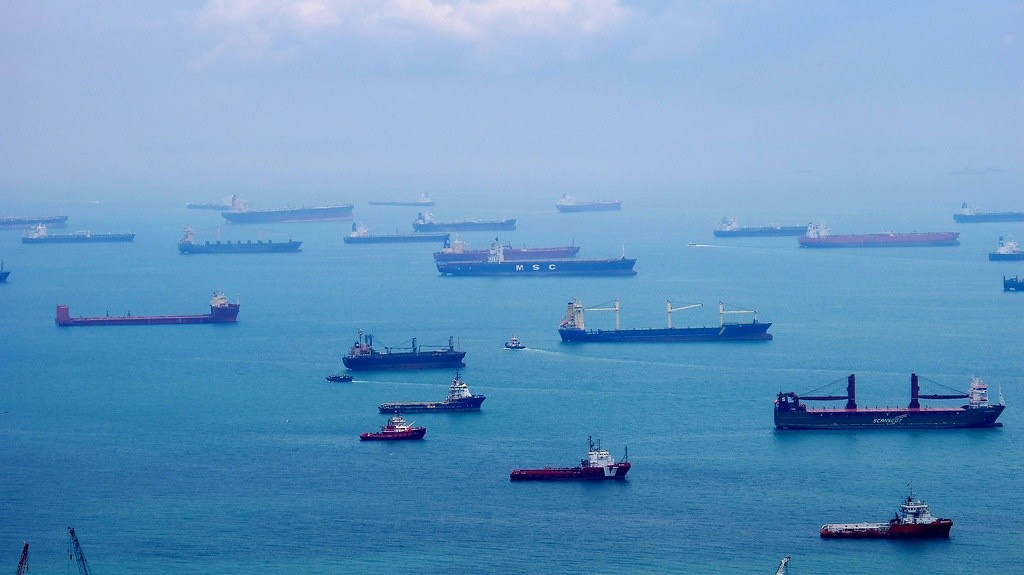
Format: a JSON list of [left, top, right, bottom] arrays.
[[987, 236, 1023, 261], [433, 234, 581, 260], [412, 210, 516, 231], [325, 371, 354, 386], [772, 371, 1008, 429], [0, 263, 12, 283], [344, 223, 450, 243], [1003, 272, 1023, 293], [504, 334, 525, 349], [222, 195, 355, 222], [820, 477, 953, 540], [57, 293, 239, 327], [508, 434, 630, 483], [1, 213, 67, 228], [797, 222, 959, 246], [378, 369, 487, 414], [954, 200, 1024, 222], [688, 241, 698, 245], [715, 214, 812, 236], [342, 330, 467, 369], [177, 225, 301, 254], [557, 296, 774, 341], [185, 193, 253, 212], [22, 222, 136, 244], [436, 236, 639, 275], [360, 415, 425, 441], [556, 192, 621, 213], [368, 192, 436, 206]]
